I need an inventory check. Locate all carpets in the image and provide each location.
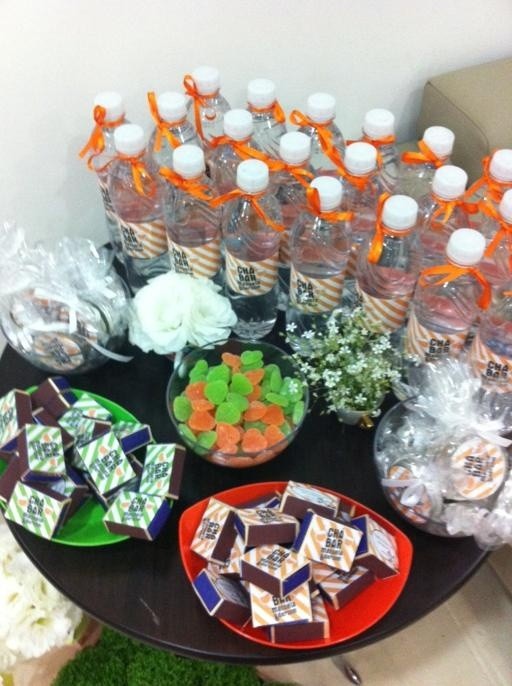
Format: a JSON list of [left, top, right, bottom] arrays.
[[52, 629, 300, 686]]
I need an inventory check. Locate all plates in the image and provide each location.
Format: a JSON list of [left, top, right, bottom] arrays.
[[178, 481, 415, 650], [1, 384, 157, 550]]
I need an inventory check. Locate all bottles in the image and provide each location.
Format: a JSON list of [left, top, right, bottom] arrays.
[[88, 68, 512, 418]]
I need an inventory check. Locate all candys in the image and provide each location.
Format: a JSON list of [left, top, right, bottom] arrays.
[[1, 273, 113, 372], [386, 432, 511, 524], [171, 352, 307, 466]]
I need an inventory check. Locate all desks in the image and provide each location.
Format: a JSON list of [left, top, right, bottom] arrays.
[[1, 241, 501, 686]]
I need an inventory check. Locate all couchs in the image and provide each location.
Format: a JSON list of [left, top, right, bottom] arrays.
[[416, 60, 511, 187]]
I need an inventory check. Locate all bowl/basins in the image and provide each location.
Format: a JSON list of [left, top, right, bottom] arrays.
[[373, 390, 510, 542], [165, 341, 312, 468]]
